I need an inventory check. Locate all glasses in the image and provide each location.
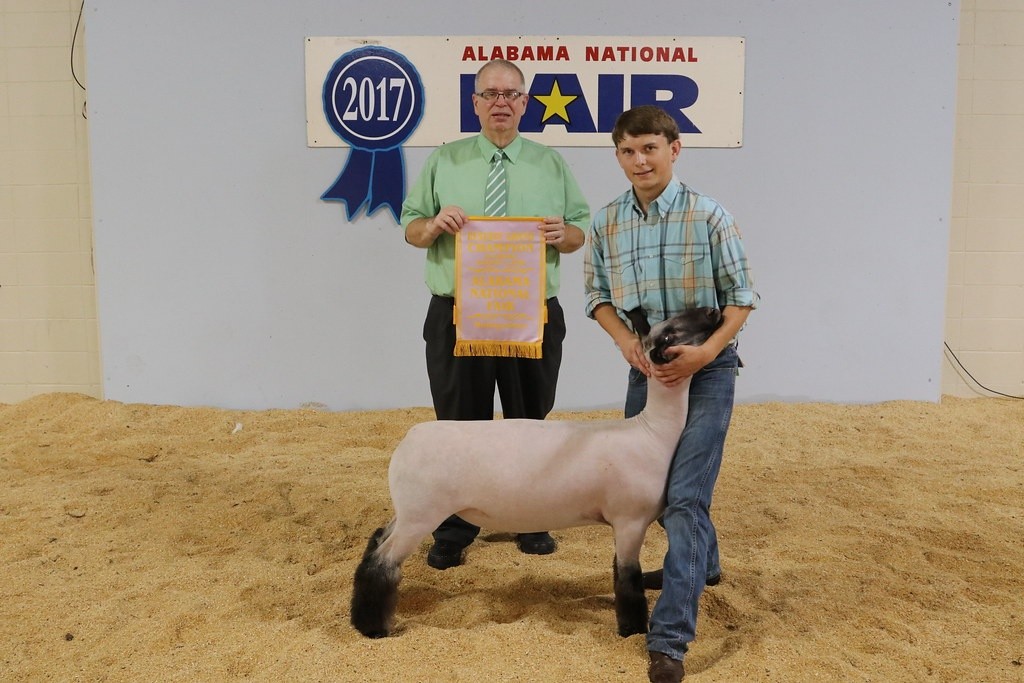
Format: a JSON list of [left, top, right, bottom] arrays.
[[476, 90, 523, 100]]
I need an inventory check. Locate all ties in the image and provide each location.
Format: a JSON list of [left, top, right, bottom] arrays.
[[485, 149, 508, 215]]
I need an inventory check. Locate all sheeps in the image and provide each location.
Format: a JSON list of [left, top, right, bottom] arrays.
[[350, 306, 723, 639]]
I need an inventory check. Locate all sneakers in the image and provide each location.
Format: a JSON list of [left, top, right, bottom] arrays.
[[648, 646, 685, 683], [515, 529, 555, 555], [426, 538, 462, 568]]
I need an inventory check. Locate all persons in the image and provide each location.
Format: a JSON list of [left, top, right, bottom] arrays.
[[585, 105, 760, 680], [401, 58, 590, 571]]
